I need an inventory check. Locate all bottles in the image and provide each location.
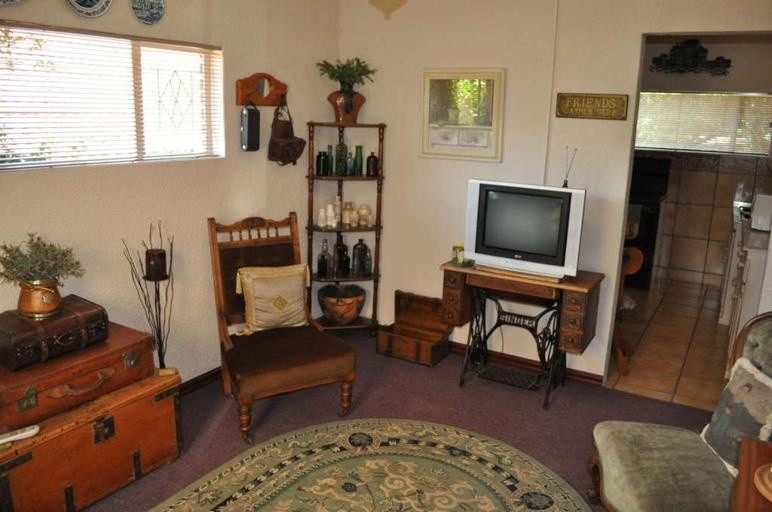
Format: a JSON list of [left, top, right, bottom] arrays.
[[447, 103, 460, 125], [316, 144, 378, 275], [452, 245, 464, 265]]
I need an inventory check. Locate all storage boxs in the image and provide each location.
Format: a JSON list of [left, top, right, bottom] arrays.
[[1, 367, 190, 511], [373, 287, 453, 368], [0, 292, 113, 367]]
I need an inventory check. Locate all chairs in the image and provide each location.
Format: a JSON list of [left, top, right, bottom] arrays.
[[209, 211, 364, 446], [589, 309, 772, 511]]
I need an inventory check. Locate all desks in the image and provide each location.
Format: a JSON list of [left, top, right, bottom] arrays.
[[722, 435, 772, 510]]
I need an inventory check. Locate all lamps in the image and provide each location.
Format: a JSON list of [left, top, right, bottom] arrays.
[[142, 246, 172, 369]]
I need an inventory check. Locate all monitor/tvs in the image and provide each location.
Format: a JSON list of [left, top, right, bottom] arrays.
[[464, 178, 586, 284]]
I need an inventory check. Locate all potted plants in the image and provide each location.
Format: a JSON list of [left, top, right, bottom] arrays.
[[315, 58, 378, 125], [0, 233, 86, 325]]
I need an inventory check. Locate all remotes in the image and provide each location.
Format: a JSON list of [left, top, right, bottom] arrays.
[[0, 425, 40, 444]]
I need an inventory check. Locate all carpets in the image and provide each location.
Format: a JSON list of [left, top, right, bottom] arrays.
[[147, 416, 595, 511], [83, 327, 713, 512]]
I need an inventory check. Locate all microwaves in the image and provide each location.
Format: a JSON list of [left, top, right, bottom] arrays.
[[749, 191, 771, 231]]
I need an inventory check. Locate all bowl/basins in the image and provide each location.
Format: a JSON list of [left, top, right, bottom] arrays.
[[319, 285, 365, 324]]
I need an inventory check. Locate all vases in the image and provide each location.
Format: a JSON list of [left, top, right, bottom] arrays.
[[317, 283, 365, 328]]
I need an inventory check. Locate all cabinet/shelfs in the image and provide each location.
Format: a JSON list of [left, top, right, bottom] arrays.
[[714, 200, 767, 380], [433, 251, 602, 410], [304, 121, 386, 340], [621, 155, 673, 292]]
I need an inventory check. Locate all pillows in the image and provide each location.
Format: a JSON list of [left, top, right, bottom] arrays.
[[230, 262, 311, 338], [699, 358, 772, 480]]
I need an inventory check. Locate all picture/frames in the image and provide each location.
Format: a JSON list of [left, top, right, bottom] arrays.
[[417, 65, 509, 165]]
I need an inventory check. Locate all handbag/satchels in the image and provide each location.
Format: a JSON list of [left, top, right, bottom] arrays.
[[267, 92, 306, 165]]
